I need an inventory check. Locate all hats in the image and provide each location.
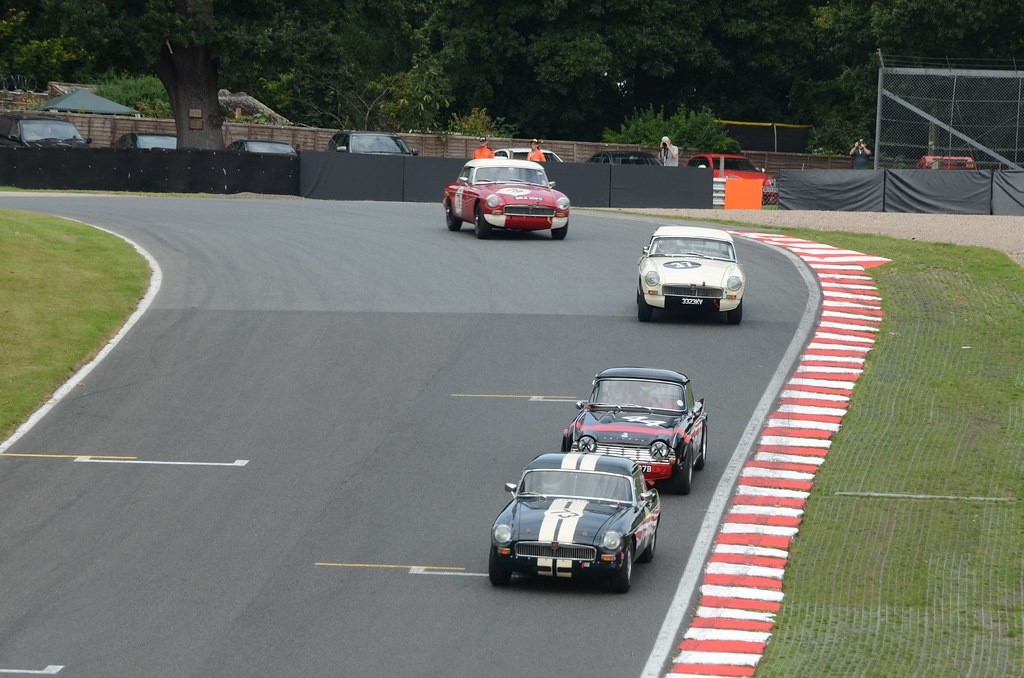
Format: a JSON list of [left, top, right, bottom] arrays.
[[479, 136, 486, 142], [530, 139, 539, 144]]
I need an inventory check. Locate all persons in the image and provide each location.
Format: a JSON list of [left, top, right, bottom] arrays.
[[847, 137, 873, 170], [658, 136, 679, 168], [526, 138, 546, 163], [474, 136, 495, 160]]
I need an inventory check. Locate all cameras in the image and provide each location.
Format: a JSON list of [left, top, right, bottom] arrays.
[[662, 143, 667, 147], [857, 141, 863, 146]]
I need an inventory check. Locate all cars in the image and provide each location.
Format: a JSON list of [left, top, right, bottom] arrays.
[[325, 132, 420, 156], [560, 368, 709, 497], [493, 148, 563, 163], [225, 139, 297, 155], [488, 452, 661, 591], [585, 151, 663, 166], [110, 133, 178, 150], [637, 225, 746, 326], [8, 117, 92, 149], [682, 155, 779, 205], [444, 156, 571, 241]]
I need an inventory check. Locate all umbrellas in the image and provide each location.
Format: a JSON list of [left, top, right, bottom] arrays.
[[36, 86, 143, 148]]
